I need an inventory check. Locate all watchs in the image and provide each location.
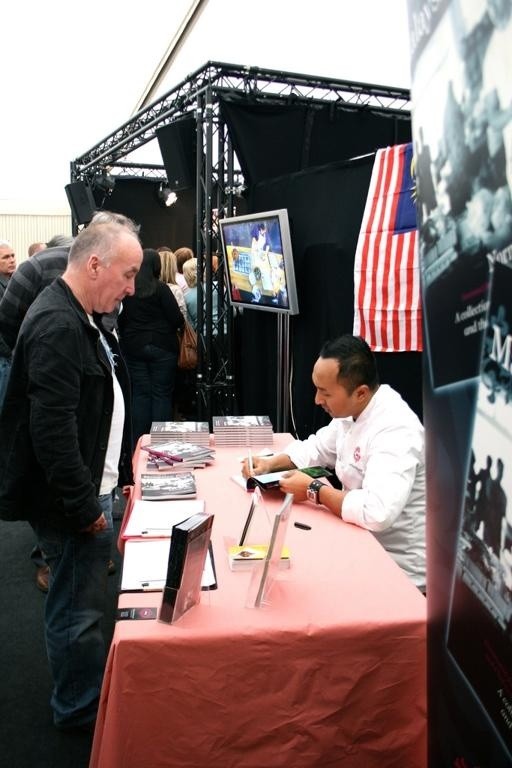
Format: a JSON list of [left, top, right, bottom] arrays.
[[307, 480, 328, 504]]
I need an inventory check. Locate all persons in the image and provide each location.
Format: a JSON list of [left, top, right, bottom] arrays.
[[464, 449, 507, 559], [242, 335, 426, 594]]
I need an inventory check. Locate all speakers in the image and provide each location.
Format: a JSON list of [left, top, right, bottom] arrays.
[[65, 181, 96, 224], [155, 118, 205, 192]]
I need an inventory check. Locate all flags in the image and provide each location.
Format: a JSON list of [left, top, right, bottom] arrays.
[[352, 141, 424, 352]]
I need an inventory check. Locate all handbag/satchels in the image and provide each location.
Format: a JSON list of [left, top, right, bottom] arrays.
[[174, 315, 200, 372]]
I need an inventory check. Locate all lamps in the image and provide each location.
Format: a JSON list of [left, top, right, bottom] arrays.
[[90, 164, 181, 207]]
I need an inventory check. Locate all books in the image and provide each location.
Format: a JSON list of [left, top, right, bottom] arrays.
[[231, 464, 333, 492], [141, 415, 275, 500]]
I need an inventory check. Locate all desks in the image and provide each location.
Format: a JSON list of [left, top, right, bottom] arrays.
[[94, 428, 434, 763]]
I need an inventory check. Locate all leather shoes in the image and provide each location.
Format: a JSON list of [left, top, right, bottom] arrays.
[[35, 563, 50, 593], [107, 558, 116, 576]]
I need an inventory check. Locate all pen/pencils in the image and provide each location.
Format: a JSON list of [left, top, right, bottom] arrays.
[[247, 445, 254, 471]]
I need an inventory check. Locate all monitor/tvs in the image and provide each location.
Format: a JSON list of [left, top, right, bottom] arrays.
[[218, 208, 300, 316]]
